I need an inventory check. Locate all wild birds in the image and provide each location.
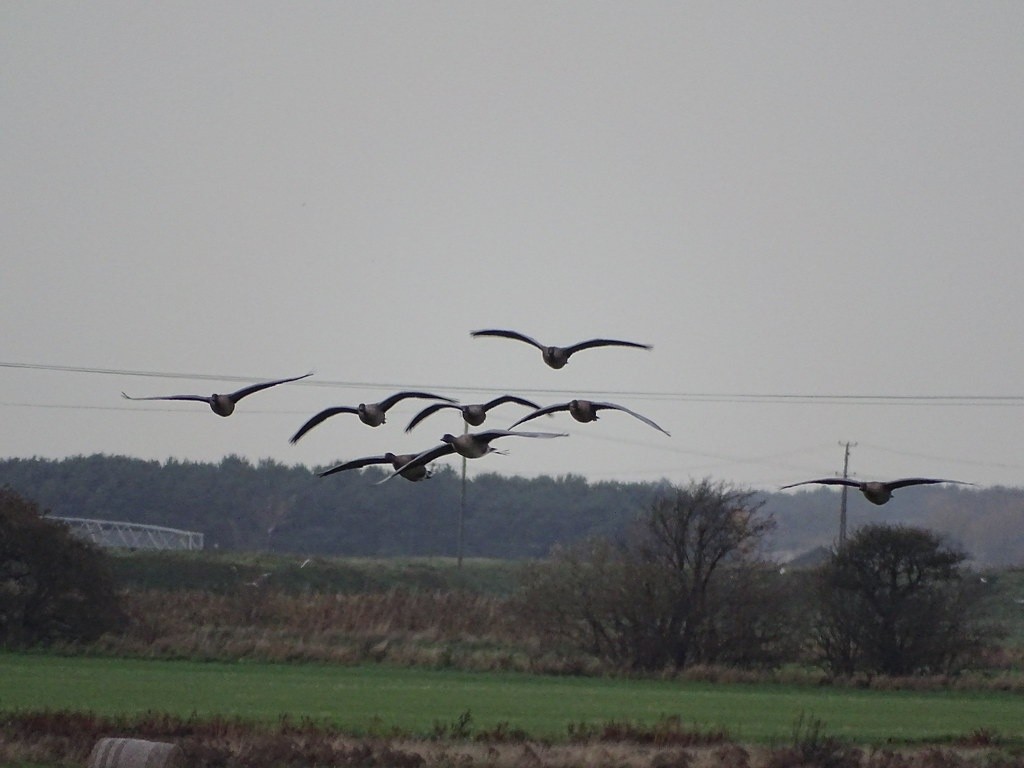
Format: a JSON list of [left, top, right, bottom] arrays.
[[375, 427, 573, 487], [119, 372, 316, 419], [286, 389, 463, 446], [506, 398, 672, 438], [315, 451, 436, 483], [404, 393, 556, 435], [469, 328, 652, 370], [779, 474, 974, 508]]
